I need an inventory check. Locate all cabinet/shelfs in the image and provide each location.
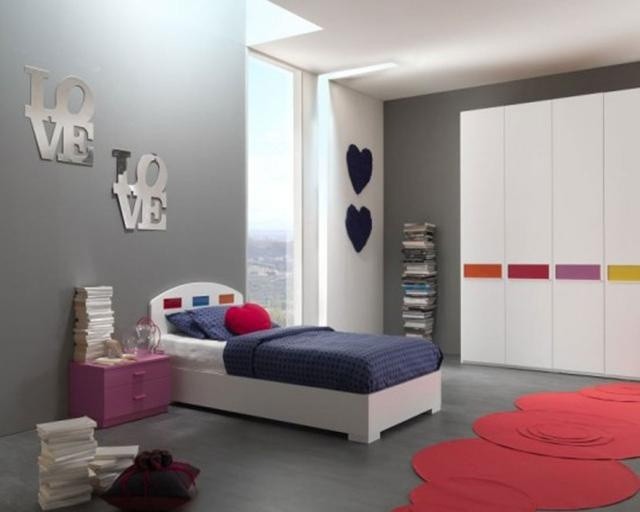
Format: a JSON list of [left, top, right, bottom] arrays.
[[460, 87, 640, 384]]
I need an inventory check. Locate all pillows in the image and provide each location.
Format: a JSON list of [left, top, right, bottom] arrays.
[[98, 449, 202, 509], [164, 300, 281, 341]]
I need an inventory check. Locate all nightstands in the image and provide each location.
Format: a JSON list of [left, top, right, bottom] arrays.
[[67, 350, 172, 430]]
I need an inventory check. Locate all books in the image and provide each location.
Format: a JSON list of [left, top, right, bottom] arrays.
[[35, 416, 140, 511], [73, 286, 115, 363], [400, 222, 438, 341]]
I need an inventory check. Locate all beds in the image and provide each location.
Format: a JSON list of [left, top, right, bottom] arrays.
[[148, 281, 443, 444]]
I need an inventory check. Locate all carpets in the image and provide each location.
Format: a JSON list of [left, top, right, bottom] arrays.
[[393, 381, 640, 512]]
